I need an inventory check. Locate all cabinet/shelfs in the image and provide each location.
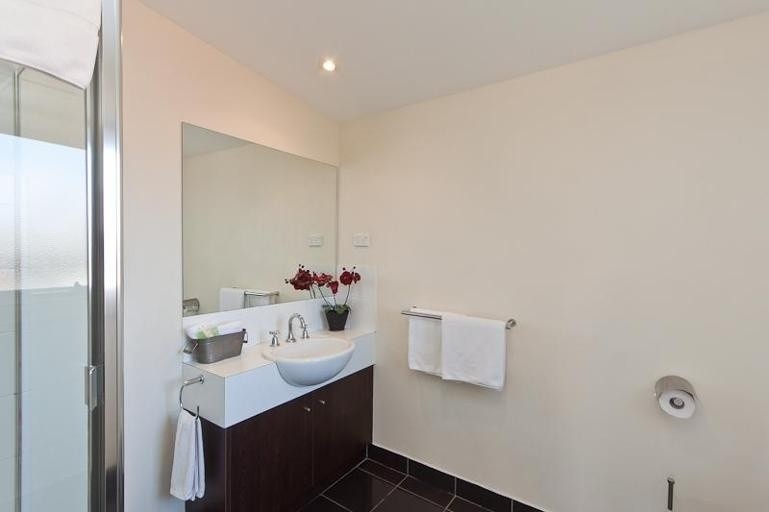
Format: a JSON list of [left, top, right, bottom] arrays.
[[188, 363, 377, 511]]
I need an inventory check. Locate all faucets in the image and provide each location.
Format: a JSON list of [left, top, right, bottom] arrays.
[[287, 312, 307, 342]]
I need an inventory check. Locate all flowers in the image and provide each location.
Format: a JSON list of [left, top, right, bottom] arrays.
[[281, 264, 320, 298], [312, 266, 365, 315]]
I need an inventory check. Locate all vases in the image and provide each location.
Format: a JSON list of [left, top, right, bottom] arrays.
[[327, 307, 349, 331]]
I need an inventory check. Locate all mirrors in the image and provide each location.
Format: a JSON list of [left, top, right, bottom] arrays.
[[180, 119, 341, 319]]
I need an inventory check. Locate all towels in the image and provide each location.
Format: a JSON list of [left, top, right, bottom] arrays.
[[407, 306, 455, 377], [167, 410, 206, 503], [246, 289, 277, 307], [216, 286, 247, 312], [439, 314, 508, 391]]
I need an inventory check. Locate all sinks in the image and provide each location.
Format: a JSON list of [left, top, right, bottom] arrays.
[[260, 338, 356, 388]]
